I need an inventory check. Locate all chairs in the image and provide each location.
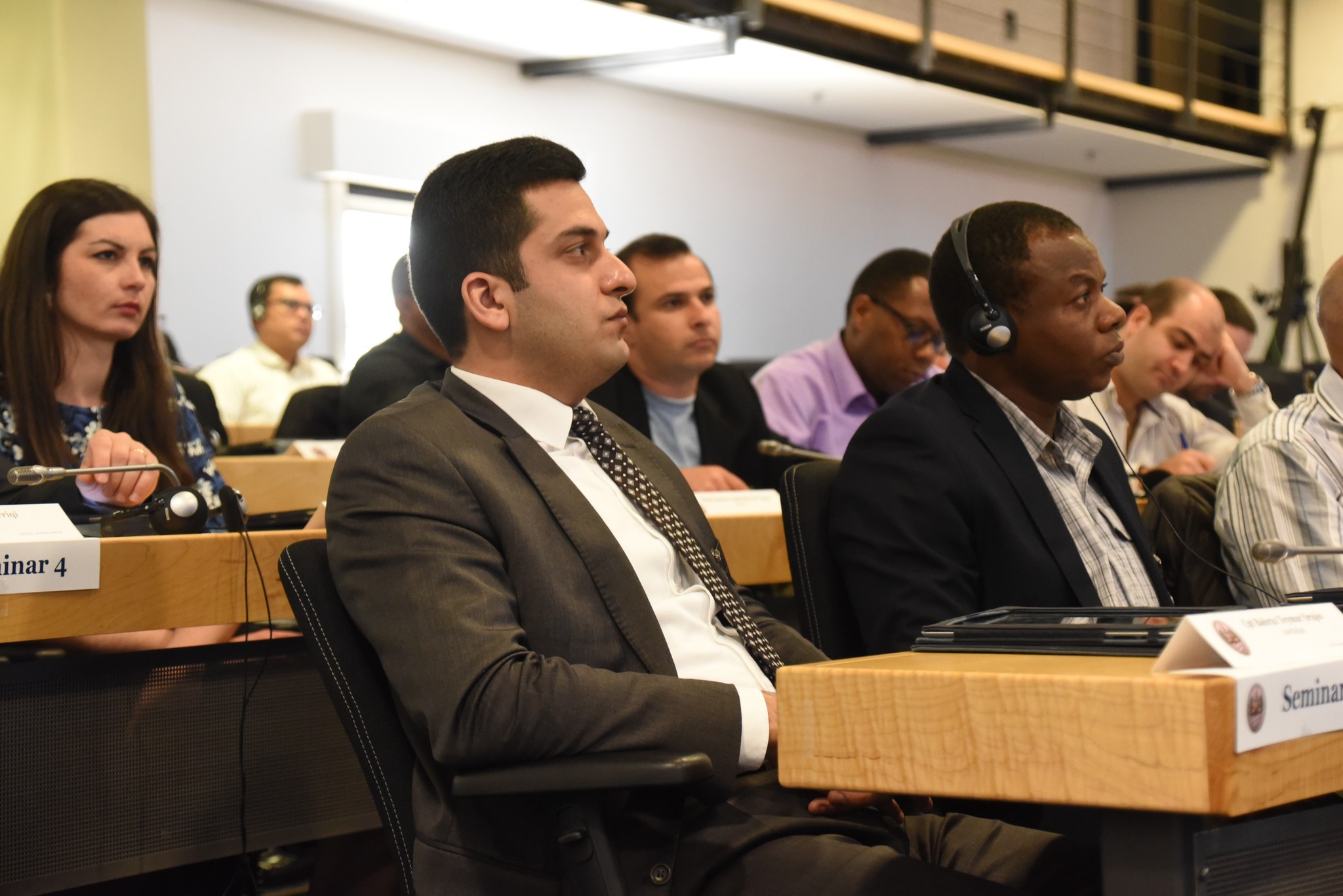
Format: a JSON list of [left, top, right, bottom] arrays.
[[275, 538, 715, 896], [780, 457, 846, 662], [1142, 469, 1236, 603], [274, 386, 347, 436]]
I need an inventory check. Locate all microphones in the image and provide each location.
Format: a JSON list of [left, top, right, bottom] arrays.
[[6, 458, 207, 536], [1251, 537, 1343, 564], [757, 438, 842, 463]]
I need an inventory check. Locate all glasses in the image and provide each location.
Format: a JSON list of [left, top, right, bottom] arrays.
[[877, 297, 945, 351]]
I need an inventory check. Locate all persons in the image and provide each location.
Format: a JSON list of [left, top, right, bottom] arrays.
[[337, 253, 451, 437], [318, 136, 1100, 896], [585, 232, 818, 512], [1212, 252, 1343, 608], [0, 177, 305, 722], [192, 275, 350, 432], [1065, 275, 1282, 499], [747, 248, 946, 476], [826, 199, 1179, 665]]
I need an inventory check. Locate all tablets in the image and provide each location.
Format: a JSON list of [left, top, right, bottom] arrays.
[[919, 603, 1241, 638]]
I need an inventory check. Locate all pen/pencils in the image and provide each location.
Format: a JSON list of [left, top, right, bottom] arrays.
[[1179, 432, 1189, 449]]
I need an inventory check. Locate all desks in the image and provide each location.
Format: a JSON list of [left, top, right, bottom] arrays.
[[0, 427, 1343, 896]]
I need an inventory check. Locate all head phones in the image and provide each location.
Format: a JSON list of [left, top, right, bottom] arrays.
[[252, 278, 270, 328], [951, 210, 1019, 358]]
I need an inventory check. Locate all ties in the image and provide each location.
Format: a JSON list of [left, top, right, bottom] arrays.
[[568, 405, 787, 689]]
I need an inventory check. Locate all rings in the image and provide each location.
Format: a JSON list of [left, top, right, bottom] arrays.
[[131, 447, 146, 457]]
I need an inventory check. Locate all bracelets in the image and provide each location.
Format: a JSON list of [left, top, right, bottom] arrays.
[[1233, 371, 1256, 389]]
